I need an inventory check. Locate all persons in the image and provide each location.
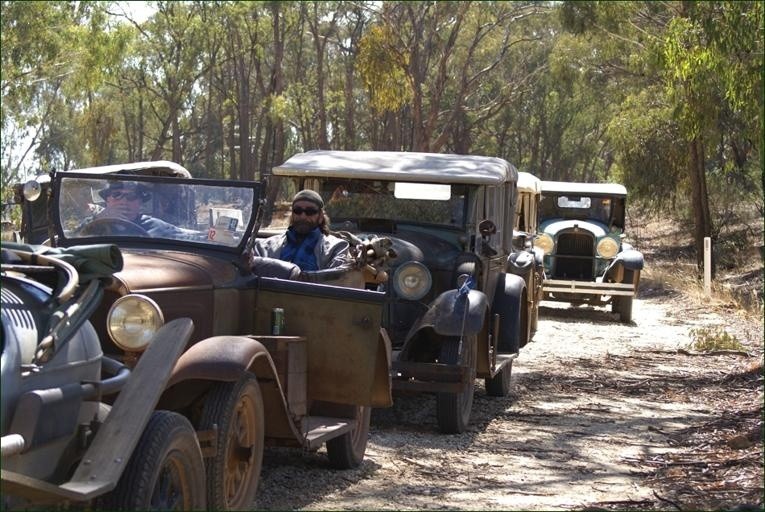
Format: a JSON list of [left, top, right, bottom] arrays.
[[35, 179, 242, 249], [241, 188, 389, 290]]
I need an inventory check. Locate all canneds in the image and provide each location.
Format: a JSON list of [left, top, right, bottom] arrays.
[[270, 306, 286, 337]]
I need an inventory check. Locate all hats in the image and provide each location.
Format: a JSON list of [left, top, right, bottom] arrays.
[[99, 170, 151, 203], [293, 189, 324, 208]]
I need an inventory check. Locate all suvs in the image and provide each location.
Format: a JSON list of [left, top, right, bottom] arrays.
[[2, 157, 394, 512]]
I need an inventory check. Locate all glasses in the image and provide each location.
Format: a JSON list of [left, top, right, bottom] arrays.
[[293, 208, 320, 216], [111, 192, 137, 201]]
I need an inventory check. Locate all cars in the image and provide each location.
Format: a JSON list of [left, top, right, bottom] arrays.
[[269, 147, 557, 439], [537, 178, 644, 324]]
